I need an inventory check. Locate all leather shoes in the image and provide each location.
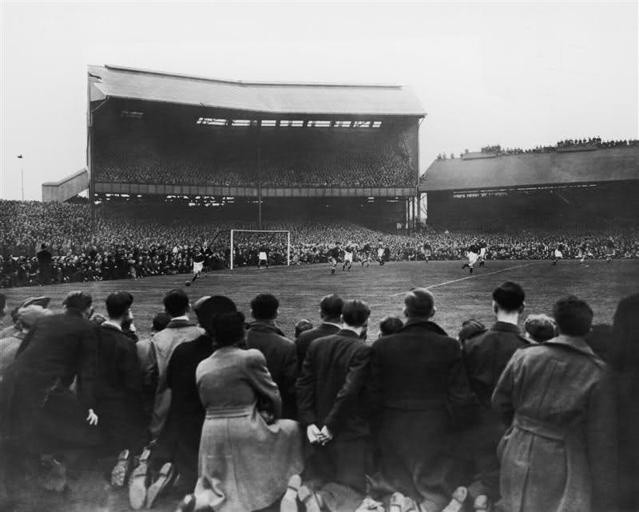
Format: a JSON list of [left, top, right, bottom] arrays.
[[298, 486, 321, 512], [441, 486, 469, 512], [473, 495, 492, 512], [280, 473, 302, 512], [111, 449, 177, 511]]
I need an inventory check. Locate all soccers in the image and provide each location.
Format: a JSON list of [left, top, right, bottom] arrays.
[[184, 280, 191, 286]]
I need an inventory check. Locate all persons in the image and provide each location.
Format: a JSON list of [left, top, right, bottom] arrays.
[[1, 199, 637, 282], [91, 135, 415, 191], [436, 135, 638, 160], [0, 281, 639, 511]]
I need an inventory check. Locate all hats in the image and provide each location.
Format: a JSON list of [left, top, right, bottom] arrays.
[[523, 314, 559, 342], [459, 319, 489, 341], [18, 290, 134, 327]]
